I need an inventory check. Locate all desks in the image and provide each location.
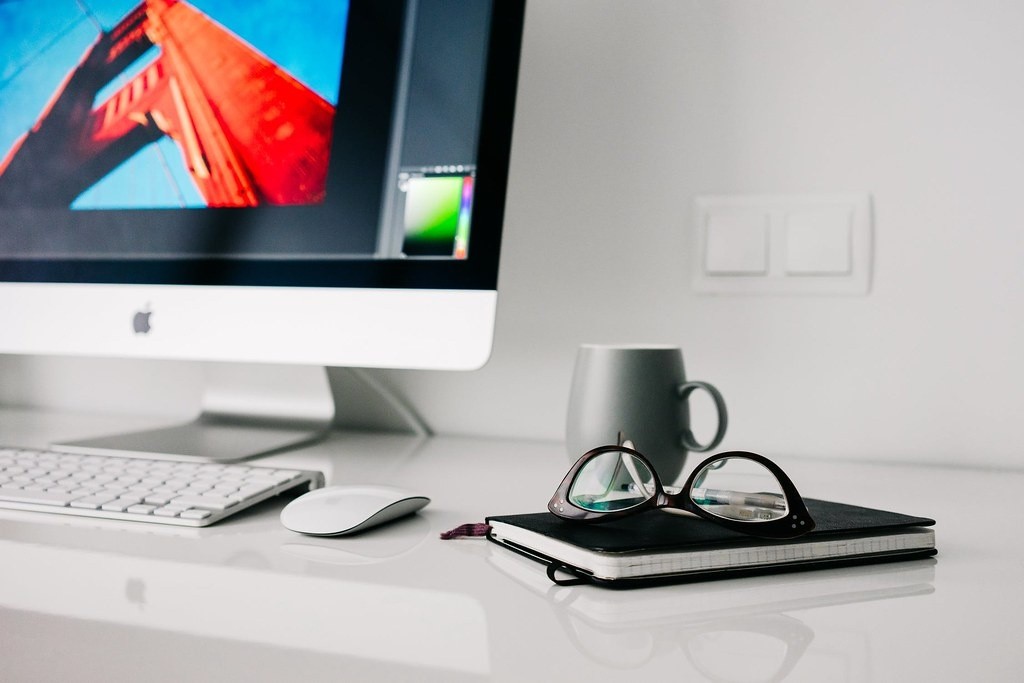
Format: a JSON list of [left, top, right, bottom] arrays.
[[0, 409, 1024, 683]]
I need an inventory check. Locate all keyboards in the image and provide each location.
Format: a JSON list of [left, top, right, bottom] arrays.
[[0, 442, 326, 528]]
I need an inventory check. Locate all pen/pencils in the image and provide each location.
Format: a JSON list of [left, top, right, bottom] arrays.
[[619, 478, 789, 511]]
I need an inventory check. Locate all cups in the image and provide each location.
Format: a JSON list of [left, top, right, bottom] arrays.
[[565, 343, 728, 490]]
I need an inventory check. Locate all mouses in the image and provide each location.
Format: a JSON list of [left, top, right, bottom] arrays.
[[280, 486, 431, 535]]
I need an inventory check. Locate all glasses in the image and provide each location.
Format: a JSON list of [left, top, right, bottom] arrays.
[[547, 430, 815, 539]]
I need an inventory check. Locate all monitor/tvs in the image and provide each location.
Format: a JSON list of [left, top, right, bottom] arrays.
[[0, 0, 533, 464]]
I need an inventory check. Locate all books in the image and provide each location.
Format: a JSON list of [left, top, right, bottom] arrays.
[[442, 489, 935, 591]]
